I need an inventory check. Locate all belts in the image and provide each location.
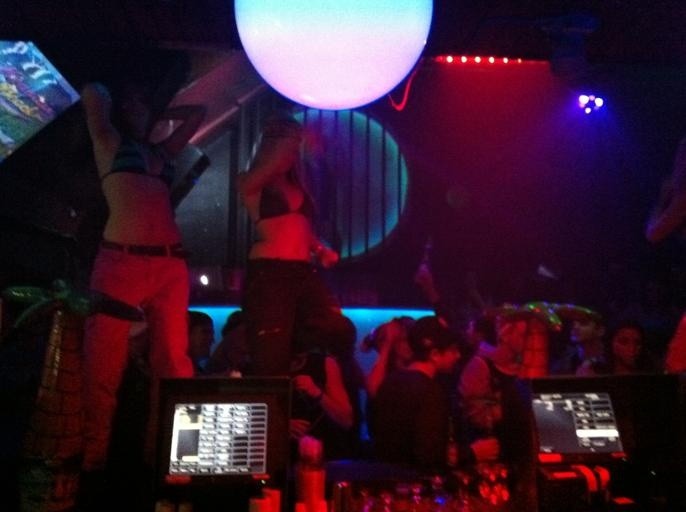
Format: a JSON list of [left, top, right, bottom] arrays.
[[331, 457, 515, 512]]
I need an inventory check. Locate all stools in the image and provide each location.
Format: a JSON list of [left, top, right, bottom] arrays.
[[98, 238, 186, 259]]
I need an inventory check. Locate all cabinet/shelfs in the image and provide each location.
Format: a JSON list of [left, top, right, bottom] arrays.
[[244, 471, 284, 511], [291, 435, 328, 511], [154, 472, 194, 511]]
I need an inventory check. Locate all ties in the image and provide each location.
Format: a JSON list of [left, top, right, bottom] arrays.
[[530, 388, 625, 462], [0, 40, 83, 166], [163, 392, 282, 486]]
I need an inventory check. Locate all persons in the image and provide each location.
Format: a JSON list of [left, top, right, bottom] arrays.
[[155, 309, 644, 511], [240, 116, 338, 373], [647, 138, 686, 371], [82, 82, 206, 469]]
